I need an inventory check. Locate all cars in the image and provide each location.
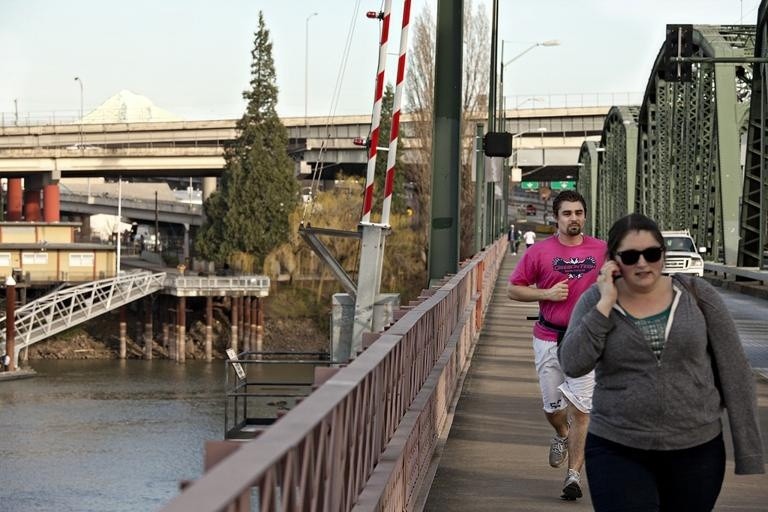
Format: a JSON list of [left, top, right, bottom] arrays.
[[295, 185, 320, 203], [516, 202, 537, 217], [542, 210, 558, 228]]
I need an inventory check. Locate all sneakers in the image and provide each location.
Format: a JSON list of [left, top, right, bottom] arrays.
[[549, 421, 571, 468], [560, 474, 582, 500]]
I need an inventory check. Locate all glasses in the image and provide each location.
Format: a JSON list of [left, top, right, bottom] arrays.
[[614, 245, 665, 265]]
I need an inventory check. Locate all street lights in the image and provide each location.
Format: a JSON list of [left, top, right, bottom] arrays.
[[73, 75, 84, 120], [500, 33, 562, 178], [302, 9, 319, 114]]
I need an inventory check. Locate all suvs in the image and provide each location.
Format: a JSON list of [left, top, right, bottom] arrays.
[[656, 229, 707, 281]]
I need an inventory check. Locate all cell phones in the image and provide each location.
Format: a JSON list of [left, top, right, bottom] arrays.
[[609, 259, 622, 283]]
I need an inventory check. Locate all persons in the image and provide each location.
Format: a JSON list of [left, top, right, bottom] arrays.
[[176, 263, 187, 277], [506, 223, 536, 256], [91, 228, 150, 256], [3, 353, 11, 372], [505, 189, 619, 501], [556, 212, 766, 512]]
